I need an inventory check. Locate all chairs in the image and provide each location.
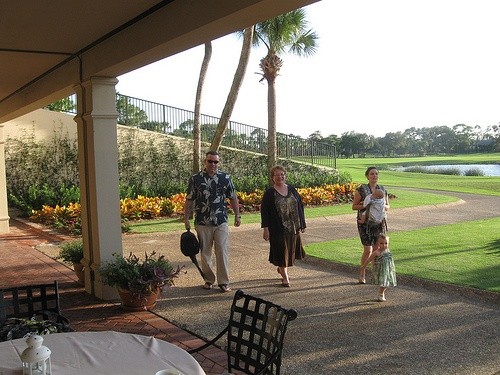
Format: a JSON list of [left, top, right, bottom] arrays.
[[0, 280, 76, 332], [188, 289, 297, 375]]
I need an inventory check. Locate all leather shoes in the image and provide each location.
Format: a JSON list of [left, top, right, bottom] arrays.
[[282, 279, 290, 287], [359, 277, 366, 284], [377, 294, 385, 302], [278, 267, 291, 281]]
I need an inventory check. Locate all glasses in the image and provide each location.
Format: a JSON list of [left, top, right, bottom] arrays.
[[206, 159, 219, 164], [273, 174, 285, 177]]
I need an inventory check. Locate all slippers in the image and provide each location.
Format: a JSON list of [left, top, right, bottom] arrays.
[[218, 283, 230, 292]]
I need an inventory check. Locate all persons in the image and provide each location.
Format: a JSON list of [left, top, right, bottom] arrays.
[[352, 167, 390, 284], [261, 166, 306, 287], [358, 189, 389, 245], [361, 234, 398, 302], [184, 151, 241, 292]]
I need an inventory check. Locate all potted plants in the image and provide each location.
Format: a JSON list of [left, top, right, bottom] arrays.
[[97, 249, 187, 311], [52, 237, 85, 282]]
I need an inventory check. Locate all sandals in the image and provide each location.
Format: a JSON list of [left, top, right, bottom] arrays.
[[203, 282, 212, 290]]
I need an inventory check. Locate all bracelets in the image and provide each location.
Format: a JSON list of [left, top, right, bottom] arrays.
[[235, 215, 241, 218]]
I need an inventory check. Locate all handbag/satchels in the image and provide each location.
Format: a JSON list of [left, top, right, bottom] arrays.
[[180, 227, 200, 257]]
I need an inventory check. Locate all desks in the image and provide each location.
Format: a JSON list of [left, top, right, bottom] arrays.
[[0, 331, 206, 375]]
